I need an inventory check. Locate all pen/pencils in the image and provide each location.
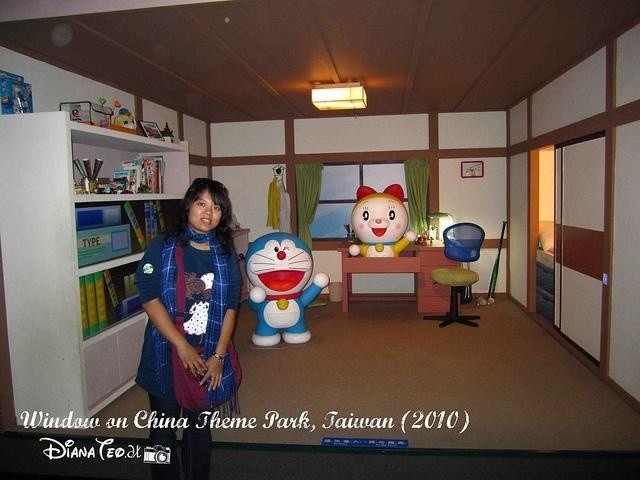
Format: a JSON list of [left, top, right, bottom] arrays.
[[344, 224, 355, 233]]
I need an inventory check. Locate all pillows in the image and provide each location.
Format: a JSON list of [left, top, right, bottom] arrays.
[[538, 230, 555, 252]]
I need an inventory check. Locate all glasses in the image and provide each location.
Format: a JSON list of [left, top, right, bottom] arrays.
[[195, 177, 225, 190]]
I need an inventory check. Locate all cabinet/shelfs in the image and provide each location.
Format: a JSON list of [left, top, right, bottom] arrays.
[[0, 109, 192, 430]]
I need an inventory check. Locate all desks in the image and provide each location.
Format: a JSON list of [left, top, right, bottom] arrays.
[[228, 228, 251, 305], [336, 239, 462, 317]]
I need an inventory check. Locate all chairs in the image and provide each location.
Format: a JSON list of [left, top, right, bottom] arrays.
[[422, 222, 485, 328]]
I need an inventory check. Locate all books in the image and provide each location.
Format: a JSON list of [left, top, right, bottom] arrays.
[[80, 270, 141, 338], [123, 202, 164, 254]]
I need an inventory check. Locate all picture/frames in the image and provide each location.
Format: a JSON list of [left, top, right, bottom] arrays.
[[138, 118, 165, 140], [461, 161, 484, 178]]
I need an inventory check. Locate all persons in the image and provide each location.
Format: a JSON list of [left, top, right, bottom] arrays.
[[347, 185, 415, 259], [136, 178, 243, 480], [245, 231, 329, 347]]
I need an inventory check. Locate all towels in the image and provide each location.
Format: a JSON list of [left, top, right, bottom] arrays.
[[266, 176, 292, 233]]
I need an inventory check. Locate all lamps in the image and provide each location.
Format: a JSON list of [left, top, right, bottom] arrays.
[[310, 82, 369, 111], [426, 212, 455, 244]]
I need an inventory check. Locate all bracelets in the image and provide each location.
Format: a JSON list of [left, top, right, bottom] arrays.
[[213, 352, 224, 362]]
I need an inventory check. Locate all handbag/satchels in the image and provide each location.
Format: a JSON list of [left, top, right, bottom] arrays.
[[171, 340, 242, 412]]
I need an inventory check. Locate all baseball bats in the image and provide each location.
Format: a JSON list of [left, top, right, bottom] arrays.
[[487, 221, 509, 304]]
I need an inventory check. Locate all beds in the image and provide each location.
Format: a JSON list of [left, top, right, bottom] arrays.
[[536, 220, 555, 270]]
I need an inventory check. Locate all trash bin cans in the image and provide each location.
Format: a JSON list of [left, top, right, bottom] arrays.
[[328, 282, 342, 303]]
